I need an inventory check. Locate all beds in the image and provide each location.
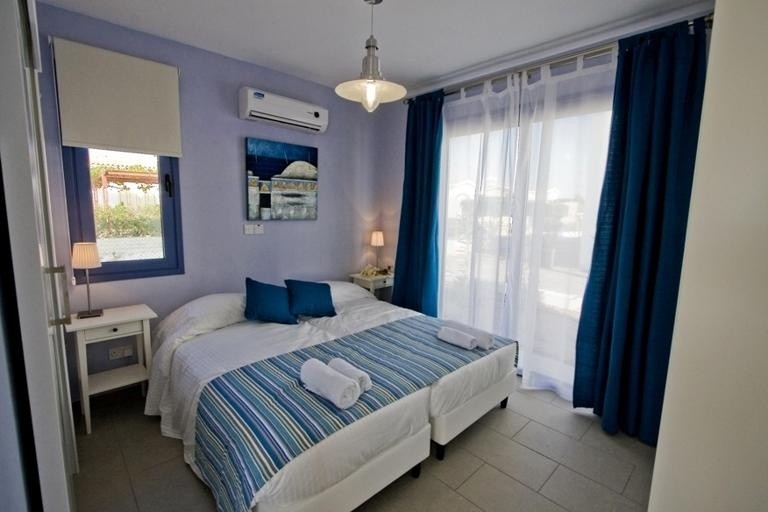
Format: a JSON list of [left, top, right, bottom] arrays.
[[144, 314, 432, 511], [287, 280, 520, 461]]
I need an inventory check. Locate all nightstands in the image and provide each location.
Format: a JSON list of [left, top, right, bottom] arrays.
[[62, 304, 158, 435], [351, 274, 394, 296]]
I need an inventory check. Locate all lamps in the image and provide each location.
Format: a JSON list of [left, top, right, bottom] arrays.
[[71, 243, 104, 319], [334, 0, 407, 112], [370, 231, 385, 274]]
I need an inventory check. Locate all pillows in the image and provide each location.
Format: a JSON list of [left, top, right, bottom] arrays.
[[244, 277, 299, 325], [284, 281, 338, 318]]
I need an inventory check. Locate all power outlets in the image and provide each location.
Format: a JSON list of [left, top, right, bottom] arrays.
[[243, 224, 265, 235]]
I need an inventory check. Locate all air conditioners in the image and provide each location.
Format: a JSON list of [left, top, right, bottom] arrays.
[[238, 86, 329, 134]]
